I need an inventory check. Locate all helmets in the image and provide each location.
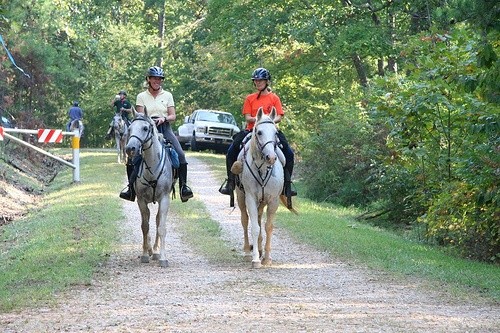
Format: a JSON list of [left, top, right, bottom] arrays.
[[251, 68, 270, 80], [119, 91, 127, 96], [148, 66, 165, 79]]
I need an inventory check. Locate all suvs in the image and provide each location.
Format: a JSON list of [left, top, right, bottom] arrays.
[[175, 109, 241, 151]]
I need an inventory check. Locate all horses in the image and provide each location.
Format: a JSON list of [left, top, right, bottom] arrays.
[[111, 109, 132, 165], [69, 118, 84, 141], [234, 104, 301, 270], [124, 103, 180, 268]]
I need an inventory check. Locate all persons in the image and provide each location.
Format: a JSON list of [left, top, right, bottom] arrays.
[[106, 91, 132, 138], [120, 66, 192, 200], [66, 102, 83, 138], [221, 68, 298, 196]]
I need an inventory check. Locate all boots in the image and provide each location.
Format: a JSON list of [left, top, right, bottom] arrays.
[[221, 155, 238, 195], [179, 162, 192, 203], [282, 154, 297, 196], [118, 157, 142, 202]]
